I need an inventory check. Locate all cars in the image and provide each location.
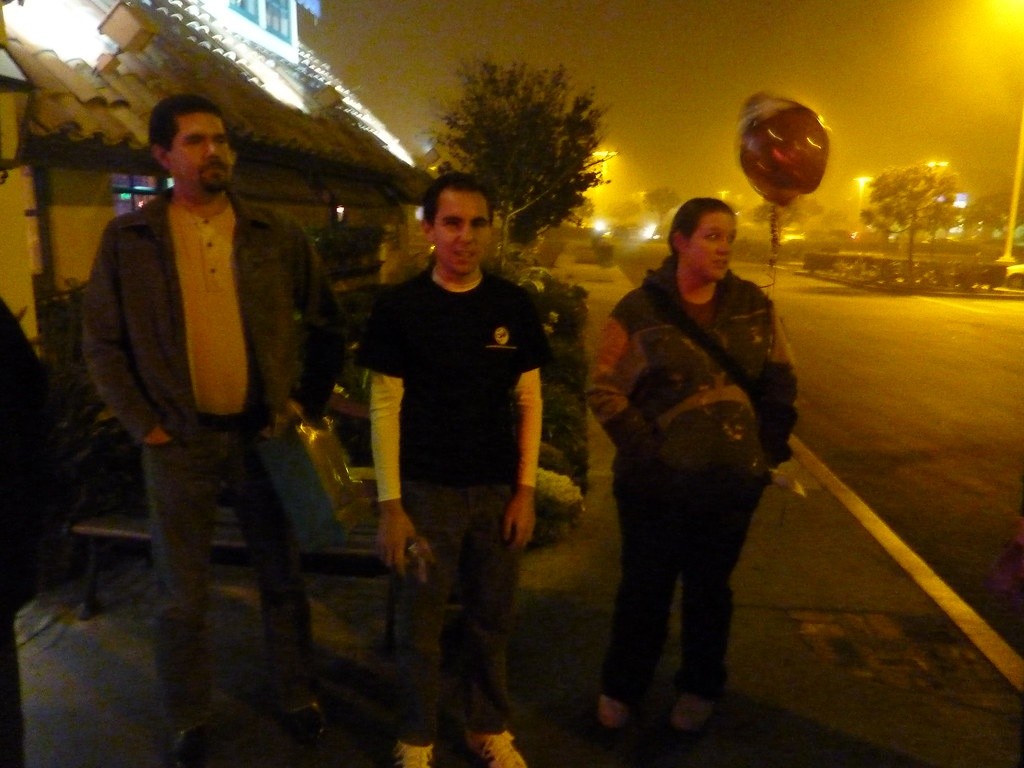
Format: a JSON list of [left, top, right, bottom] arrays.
[[1004, 264, 1024, 288]]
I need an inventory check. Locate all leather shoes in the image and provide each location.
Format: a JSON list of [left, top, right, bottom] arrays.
[[284, 699, 324, 745], [167, 724, 209, 768]]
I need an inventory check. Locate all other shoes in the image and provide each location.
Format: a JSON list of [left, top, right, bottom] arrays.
[[599, 695, 627, 727], [671, 694, 714, 731]]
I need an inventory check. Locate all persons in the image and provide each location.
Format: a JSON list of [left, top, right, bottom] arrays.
[[588, 197, 800, 751], [0, 299, 55, 768], [352, 171, 552, 768], [84, 94, 347, 768]]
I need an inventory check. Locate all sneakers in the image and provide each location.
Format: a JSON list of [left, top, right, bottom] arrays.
[[464, 728, 527, 768], [392, 740, 433, 768]]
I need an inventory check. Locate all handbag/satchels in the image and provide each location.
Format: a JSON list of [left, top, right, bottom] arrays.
[[751, 386, 794, 467]]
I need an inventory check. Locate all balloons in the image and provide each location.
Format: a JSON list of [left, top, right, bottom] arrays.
[[738, 92, 830, 208]]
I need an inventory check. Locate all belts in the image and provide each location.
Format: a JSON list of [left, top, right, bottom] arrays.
[[195, 411, 271, 434]]
